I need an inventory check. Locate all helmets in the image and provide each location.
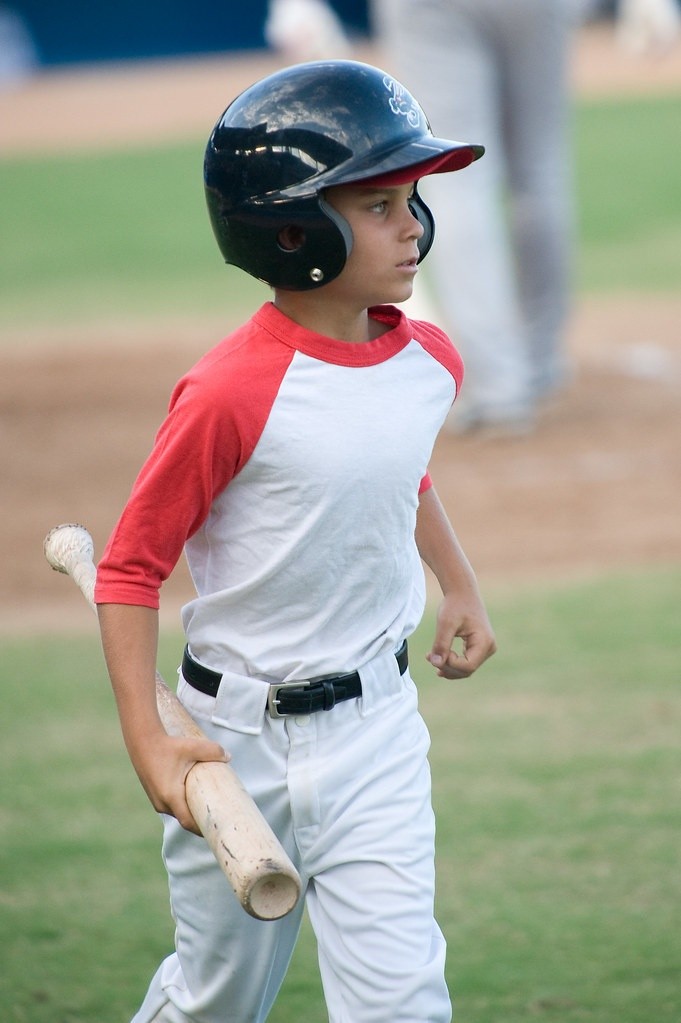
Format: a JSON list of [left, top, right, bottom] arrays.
[[202, 58, 486, 291]]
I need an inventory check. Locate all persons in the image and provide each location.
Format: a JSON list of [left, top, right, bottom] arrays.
[[95, 57, 498, 1023], [373, 1, 580, 434]]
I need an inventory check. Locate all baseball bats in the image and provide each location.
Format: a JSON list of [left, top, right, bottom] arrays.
[[41, 523, 302, 923]]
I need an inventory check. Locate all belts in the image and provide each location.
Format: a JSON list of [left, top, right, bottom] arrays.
[[181, 638, 409, 719]]
